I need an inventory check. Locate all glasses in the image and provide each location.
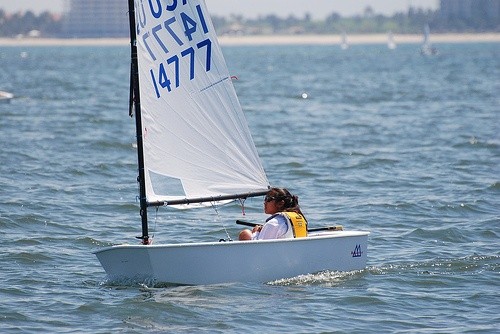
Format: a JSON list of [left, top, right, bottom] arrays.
[[265, 195, 285, 202]]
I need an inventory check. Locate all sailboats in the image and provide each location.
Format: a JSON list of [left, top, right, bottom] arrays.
[[93, 0, 370, 286]]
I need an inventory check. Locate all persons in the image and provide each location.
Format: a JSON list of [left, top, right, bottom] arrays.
[[239, 187, 308, 241]]
